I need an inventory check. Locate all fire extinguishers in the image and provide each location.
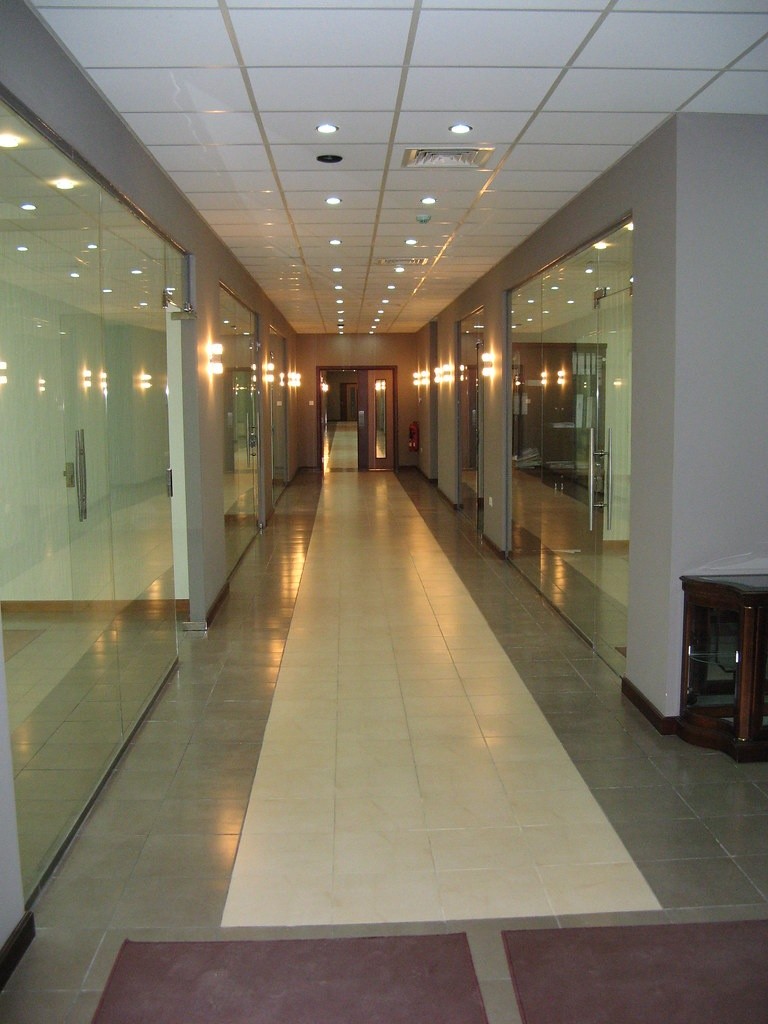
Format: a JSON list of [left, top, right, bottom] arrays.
[[408, 421, 419, 452]]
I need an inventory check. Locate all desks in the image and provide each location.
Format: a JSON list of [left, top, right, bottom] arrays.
[[676, 574, 768, 765]]
